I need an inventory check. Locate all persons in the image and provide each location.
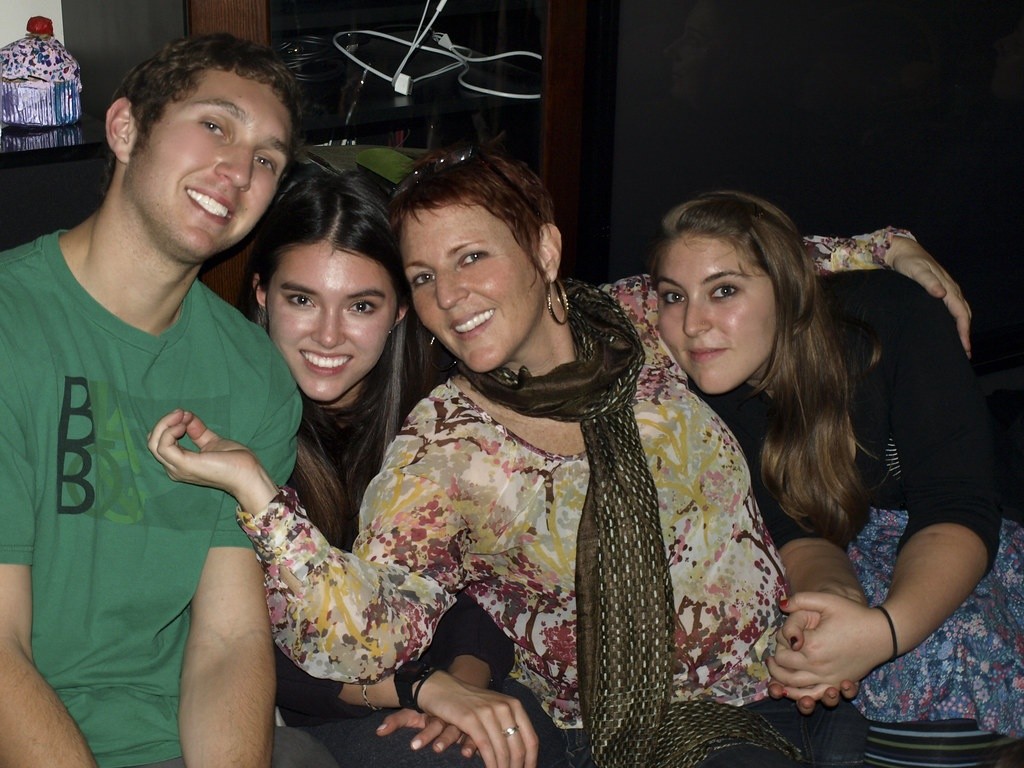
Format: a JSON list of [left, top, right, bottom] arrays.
[[235, 159, 567, 768], [146, 147, 971, 768], [0, 32, 335, 768], [651, 191, 1024, 768]]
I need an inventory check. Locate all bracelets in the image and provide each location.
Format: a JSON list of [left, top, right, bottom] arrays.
[[873, 604, 898, 666], [414, 667, 441, 714], [361, 683, 380, 711]]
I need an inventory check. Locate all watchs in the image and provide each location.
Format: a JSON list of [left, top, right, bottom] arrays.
[[394, 661, 427, 710]]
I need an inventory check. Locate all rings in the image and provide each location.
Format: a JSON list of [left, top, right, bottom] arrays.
[[502, 723, 519, 738]]
